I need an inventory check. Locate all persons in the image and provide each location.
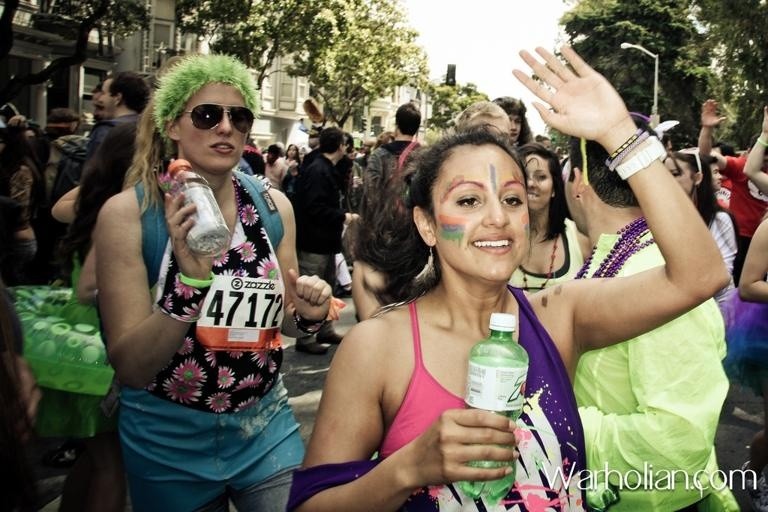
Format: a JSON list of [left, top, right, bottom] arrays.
[[0, 46, 768, 512]]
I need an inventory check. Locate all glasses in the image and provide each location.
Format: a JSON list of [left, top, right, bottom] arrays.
[[184, 104, 254, 134]]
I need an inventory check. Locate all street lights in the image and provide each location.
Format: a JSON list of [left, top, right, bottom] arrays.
[[619, 41, 663, 126]]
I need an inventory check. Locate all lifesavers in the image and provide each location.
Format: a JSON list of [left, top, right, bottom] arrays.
[[6, 286, 116, 396]]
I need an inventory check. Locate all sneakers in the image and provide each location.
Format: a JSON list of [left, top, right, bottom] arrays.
[[295, 342, 330, 354], [316, 321, 343, 344]]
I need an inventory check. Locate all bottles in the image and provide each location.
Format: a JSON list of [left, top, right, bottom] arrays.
[[454, 310, 533, 503], [167, 158, 233, 258]]
